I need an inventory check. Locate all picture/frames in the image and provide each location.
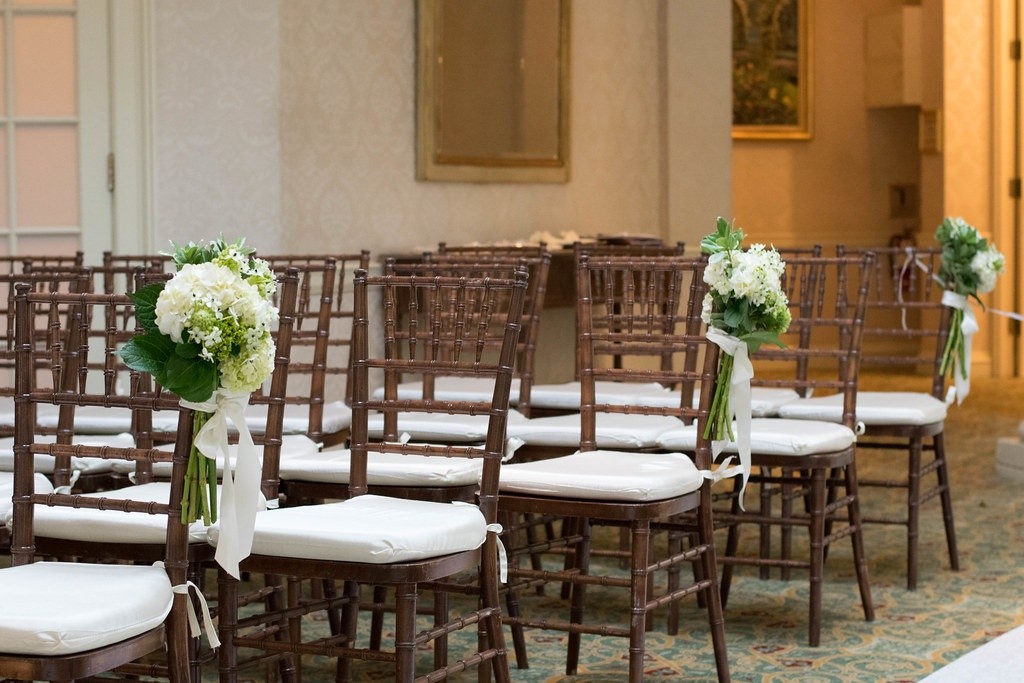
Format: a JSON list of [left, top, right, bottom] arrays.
[[731, 0, 813, 141]]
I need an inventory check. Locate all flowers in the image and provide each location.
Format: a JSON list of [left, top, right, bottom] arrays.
[[897, 216, 1023, 408], [701, 215, 792, 512], [111, 231, 280, 582]]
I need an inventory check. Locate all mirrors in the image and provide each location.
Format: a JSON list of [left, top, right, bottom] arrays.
[[412, 0, 574, 184]]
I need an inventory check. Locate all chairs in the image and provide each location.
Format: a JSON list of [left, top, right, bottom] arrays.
[[0, 242, 956, 683]]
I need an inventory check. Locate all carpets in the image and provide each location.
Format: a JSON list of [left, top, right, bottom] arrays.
[[134, 371, 1024, 683]]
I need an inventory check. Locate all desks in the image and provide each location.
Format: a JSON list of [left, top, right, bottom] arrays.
[[380, 247, 679, 385]]
